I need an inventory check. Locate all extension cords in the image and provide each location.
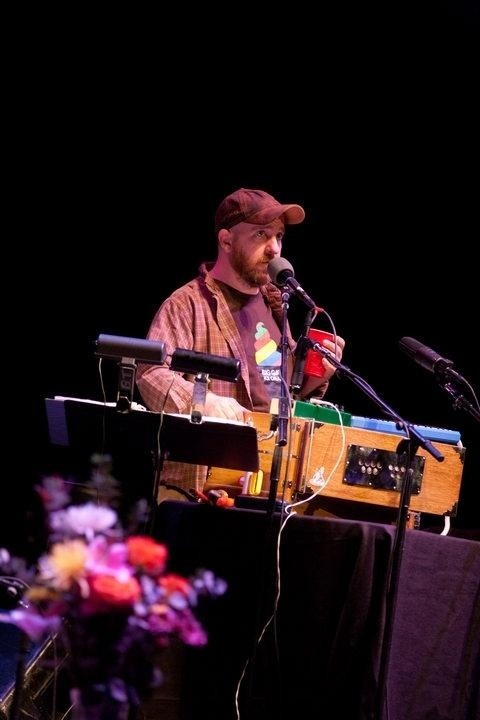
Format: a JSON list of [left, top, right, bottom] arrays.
[[236, 495, 290, 512]]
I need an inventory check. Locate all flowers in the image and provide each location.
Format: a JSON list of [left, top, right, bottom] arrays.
[[0, 476, 229, 720]]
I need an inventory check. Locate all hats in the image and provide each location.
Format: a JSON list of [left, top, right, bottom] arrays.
[[215, 188, 305, 230]]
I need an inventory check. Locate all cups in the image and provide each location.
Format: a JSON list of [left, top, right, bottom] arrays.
[[304, 327, 336, 378]]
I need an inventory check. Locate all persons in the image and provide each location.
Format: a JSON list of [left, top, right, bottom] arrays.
[[134, 187, 348, 504]]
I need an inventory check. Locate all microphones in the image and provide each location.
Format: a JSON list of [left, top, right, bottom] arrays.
[[396, 335, 454, 386], [266, 255, 322, 314], [298, 334, 321, 353]]
